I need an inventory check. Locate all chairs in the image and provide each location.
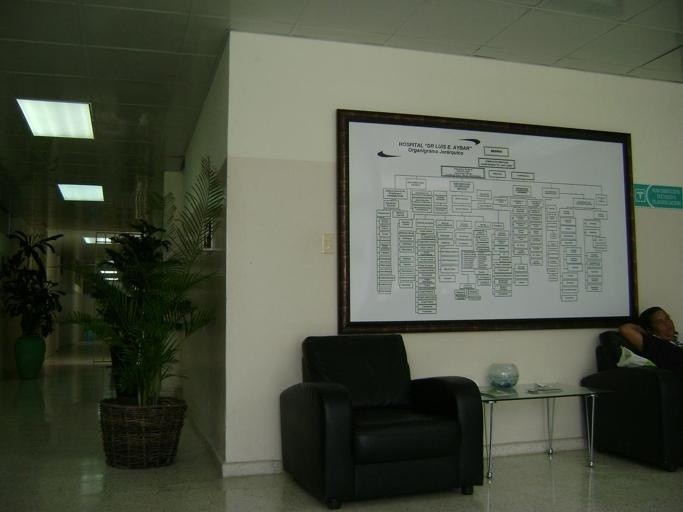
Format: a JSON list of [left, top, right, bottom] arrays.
[[280, 334, 483, 509], [582, 332, 683, 472]]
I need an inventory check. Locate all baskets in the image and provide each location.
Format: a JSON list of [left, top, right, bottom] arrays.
[[99, 396, 187, 470]]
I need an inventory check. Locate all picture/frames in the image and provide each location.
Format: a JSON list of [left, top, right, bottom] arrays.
[[336, 108, 638, 334]]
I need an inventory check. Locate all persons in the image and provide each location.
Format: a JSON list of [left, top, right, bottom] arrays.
[[619, 305, 683, 369]]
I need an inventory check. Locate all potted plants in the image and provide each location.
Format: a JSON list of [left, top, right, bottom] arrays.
[[1, 225, 67, 379], [74, 159, 226, 466]]
